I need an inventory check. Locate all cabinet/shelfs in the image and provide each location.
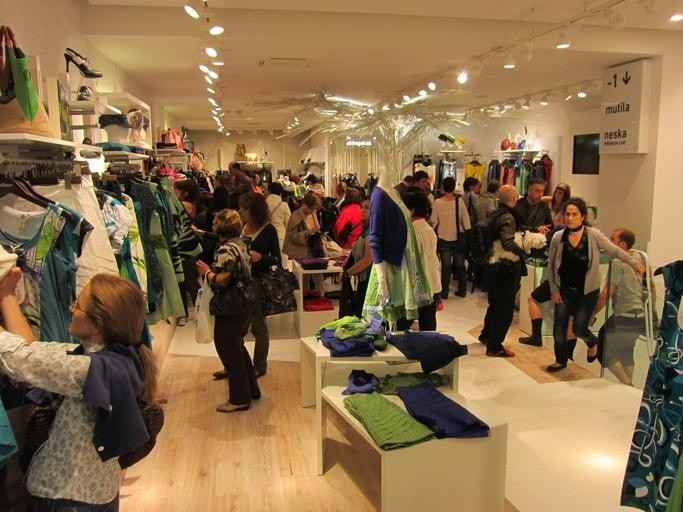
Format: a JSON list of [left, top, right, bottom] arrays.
[[96, 93, 153, 149], [242, 258, 344, 339], [519, 253, 610, 336], [301, 337, 506, 511]]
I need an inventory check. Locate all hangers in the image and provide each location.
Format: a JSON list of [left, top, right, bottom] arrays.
[[439, 156, 457, 165], [415, 155, 434, 166], [0, 153, 145, 231], [463, 153, 485, 165], [488, 153, 552, 164]]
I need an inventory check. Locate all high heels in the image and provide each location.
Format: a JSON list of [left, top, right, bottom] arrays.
[[63, 46, 104, 80]]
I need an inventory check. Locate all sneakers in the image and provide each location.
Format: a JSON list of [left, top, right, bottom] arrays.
[[517, 335, 542, 346], [253, 369, 265, 378], [478, 334, 487, 346], [485, 348, 515, 357], [212, 369, 228, 378]]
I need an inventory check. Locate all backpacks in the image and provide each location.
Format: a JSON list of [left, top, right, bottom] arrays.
[[469, 216, 504, 277]]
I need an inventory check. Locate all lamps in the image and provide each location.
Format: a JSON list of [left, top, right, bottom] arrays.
[[183, 0, 230, 136]]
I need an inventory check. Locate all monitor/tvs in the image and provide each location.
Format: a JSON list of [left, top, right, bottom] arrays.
[[572, 133, 600, 174]]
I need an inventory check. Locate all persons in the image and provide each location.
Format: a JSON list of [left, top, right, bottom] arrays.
[[393, 172, 657, 388], [286, 179, 364, 290], [174, 159, 293, 412], [361, 171, 434, 332], [0, 266, 159, 512]]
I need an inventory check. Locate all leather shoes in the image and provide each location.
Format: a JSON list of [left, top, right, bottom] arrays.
[[215, 399, 250, 412], [252, 392, 260, 399], [586, 342, 598, 363], [546, 361, 567, 372]]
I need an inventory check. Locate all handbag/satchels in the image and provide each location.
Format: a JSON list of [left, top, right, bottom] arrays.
[[18, 401, 164, 478], [0, 47, 16, 105], [456, 232, 474, 255], [467, 205, 477, 227], [208, 279, 263, 316], [5, 44, 39, 122], [499, 139, 511, 150], [258, 270, 300, 316], [338, 223, 353, 242], [510, 142, 517, 150]]
